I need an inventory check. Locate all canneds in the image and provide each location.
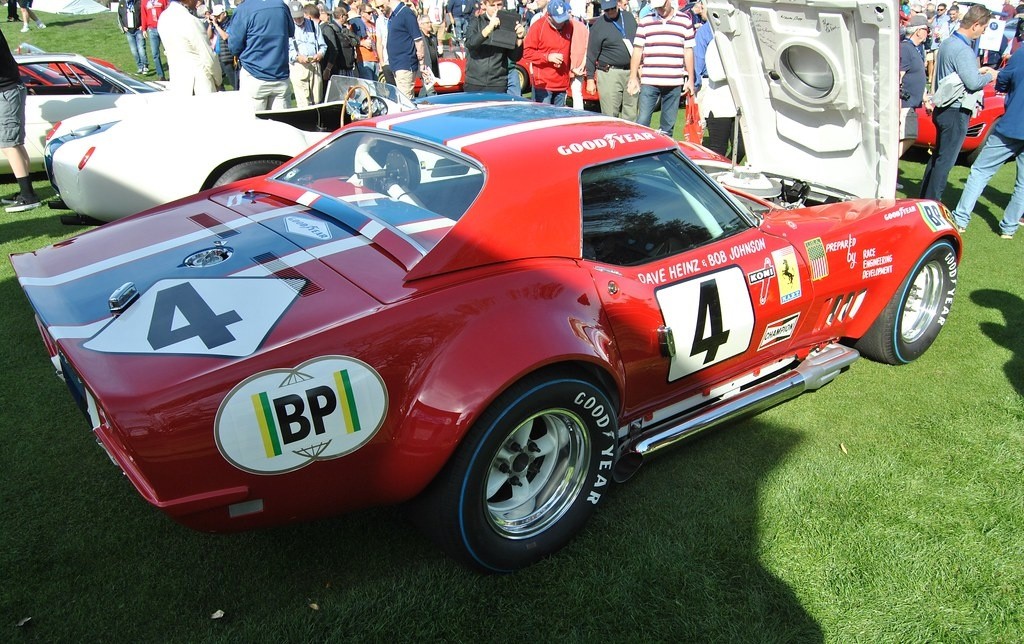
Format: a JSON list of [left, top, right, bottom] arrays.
[[553, 54, 563, 68]]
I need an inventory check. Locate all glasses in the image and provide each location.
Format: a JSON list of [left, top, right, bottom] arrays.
[[971, 11, 992, 24], [376, 5, 385, 9], [343, 14, 348, 18], [366, 11, 374, 15]]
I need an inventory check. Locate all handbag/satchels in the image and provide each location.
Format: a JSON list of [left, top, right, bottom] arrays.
[[683, 92, 704, 147]]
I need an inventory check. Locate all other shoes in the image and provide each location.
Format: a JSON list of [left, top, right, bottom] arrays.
[[21, 26, 31, 33], [1000, 234, 1014, 241], [134, 69, 143, 76], [956, 225, 966, 233], [36, 21, 46, 29], [142, 65, 149, 75]]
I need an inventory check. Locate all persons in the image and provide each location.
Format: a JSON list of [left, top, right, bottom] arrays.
[[0, 0, 1024, 239]]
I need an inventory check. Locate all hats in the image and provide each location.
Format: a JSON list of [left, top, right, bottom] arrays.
[[600, 0, 617, 10], [288, 1, 304, 18], [211, 4, 225, 16], [649, 0, 666, 10], [906, 15, 933, 28], [547, 0, 570, 24], [316, 4, 333, 15]]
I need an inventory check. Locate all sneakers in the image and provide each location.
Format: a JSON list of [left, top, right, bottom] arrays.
[[6, 200, 42, 213], [1, 192, 21, 204]]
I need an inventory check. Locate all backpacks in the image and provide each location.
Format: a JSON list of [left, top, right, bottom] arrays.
[[321, 22, 354, 71]]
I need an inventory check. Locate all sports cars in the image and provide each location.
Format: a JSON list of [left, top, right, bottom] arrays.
[[43, 75, 535, 225], [8, 0, 963, 583], [1, 43, 196, 178], [914, 16, 1020, 170]]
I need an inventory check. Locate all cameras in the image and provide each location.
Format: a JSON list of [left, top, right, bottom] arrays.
[[900, 89, 910, 101]]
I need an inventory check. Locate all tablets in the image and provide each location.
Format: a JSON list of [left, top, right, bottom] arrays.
[[489, 10, 521, 49]]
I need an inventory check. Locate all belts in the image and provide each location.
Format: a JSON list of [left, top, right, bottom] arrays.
[[610, 64, 630, 70]]
